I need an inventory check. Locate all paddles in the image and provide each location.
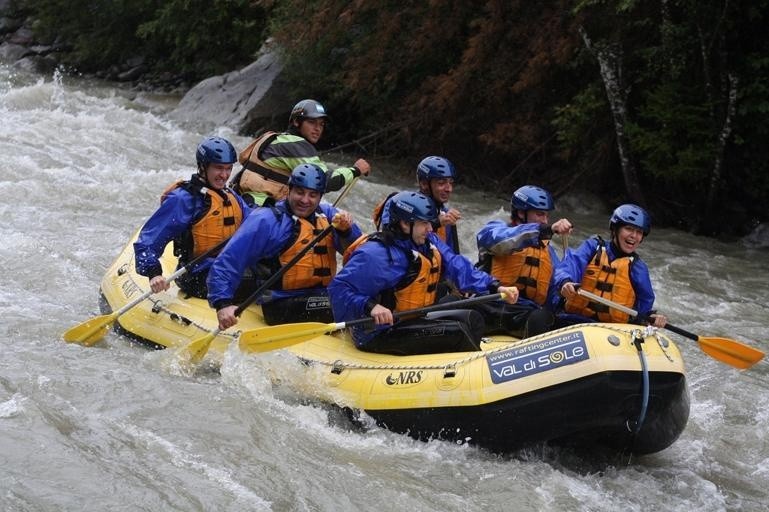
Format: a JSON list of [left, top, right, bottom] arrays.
[[62, 235, 235, 346], [239, 293, 505, 356], [574, 284, 763, 371], [177, 223, 339, 361]]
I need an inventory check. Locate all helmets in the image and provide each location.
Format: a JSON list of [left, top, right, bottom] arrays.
[[511, 185, 554, 219], [289, 99, 327, 124], [288, 163, 326, 192], [390, 157, 457, 224], [196, 137, 237, 169], [609, 204, 651, 236]]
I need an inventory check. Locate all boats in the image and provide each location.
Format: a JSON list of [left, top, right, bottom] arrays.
[[100, 211, 691, 465]]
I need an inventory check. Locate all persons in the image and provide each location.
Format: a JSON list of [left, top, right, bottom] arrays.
[[475, 186, 570, 311], [375, 155, 461, 264], [546, 202, 667, 332], [329, 193, 520, 352], [135, 137, 265, 302], [207, 164, 361, 330], [235, 97, 369, 222]]
